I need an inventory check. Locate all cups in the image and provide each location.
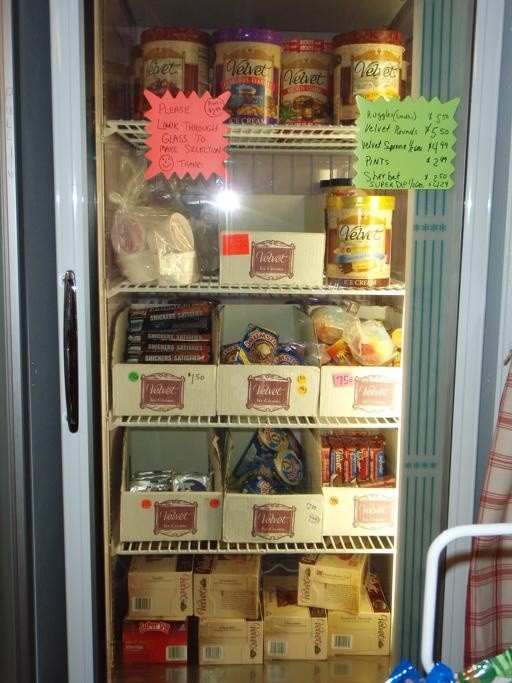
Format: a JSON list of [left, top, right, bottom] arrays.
[[112, 214, 197, 288]]
